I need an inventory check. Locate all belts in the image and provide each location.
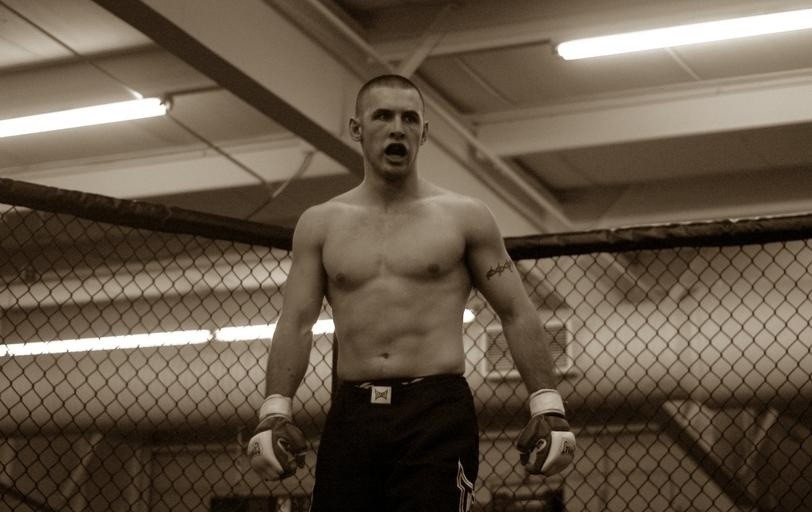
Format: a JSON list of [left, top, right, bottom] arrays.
[[338, 376, 442, 403]]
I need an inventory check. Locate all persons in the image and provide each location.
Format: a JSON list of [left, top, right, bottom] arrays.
[[245, 74, 576, 512]]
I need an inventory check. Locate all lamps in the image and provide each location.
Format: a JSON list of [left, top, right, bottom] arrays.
[[1, 99, 172, 138], [556, 10, 811, 61]]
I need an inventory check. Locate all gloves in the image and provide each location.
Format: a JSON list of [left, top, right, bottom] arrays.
[[514, 389, 576, 477], [249, 394, 306, 481]]
